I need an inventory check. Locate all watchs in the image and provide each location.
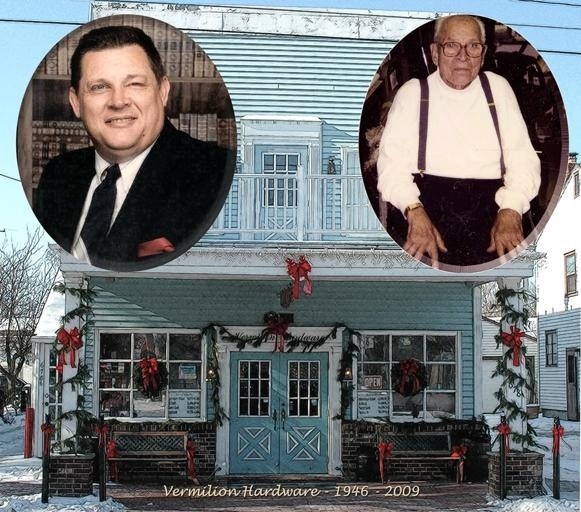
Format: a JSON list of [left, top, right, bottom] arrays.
[[403, 202, 423, 218]]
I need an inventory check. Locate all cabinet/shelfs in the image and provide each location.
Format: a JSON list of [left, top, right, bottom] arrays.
[[15, 13, 237, 213]]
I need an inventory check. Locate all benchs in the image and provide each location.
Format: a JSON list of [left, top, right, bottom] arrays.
[[380, 431, 465, 485], [106, 431, 190, 486]]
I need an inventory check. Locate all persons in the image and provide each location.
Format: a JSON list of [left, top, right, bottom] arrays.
[[376, 15, 541, 272], [31, 25, 228, 267]]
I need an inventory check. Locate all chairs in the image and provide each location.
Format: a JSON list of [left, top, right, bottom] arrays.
[[378, 85, 540, 239]]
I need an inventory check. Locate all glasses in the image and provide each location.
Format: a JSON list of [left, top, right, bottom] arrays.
[[436, 40, 486, 59]]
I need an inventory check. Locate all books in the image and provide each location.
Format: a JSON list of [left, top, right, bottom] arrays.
[[30, 13, 236, 196]]
